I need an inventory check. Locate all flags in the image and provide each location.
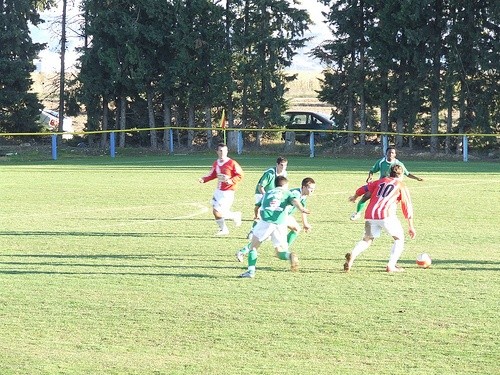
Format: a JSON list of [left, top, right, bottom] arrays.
[[219, 110, 226, 130]]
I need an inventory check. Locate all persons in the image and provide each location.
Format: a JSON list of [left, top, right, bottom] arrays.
[[247, 158, 288, 238], [343, 164, 416, 272], [350, 145, 425, 221], [198, 144, 242, 235], [238, 175, 311, 278], [236, 177, 315, 262]]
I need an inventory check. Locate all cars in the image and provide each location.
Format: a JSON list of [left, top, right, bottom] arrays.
[[39, 109, 73, 139], [280, 111, 334, 142]]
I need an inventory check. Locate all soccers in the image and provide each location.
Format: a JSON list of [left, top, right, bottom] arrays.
[[415, 252, 432, 268]]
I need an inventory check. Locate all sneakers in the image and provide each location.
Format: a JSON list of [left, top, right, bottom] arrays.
[[215, 211, 405, 279]]
